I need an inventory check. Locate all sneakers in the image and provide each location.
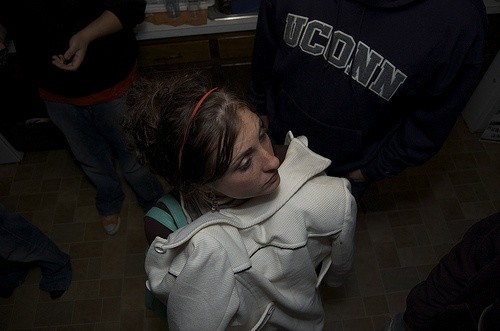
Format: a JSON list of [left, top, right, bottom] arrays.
[[100, 213, 121, 236]]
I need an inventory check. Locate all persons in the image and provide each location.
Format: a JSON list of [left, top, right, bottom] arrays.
[[0, 0, 165, 236], [0, 207, 72, 302], [384, 211, 500, 331], [123, 72, 358, 330], [252, 0, 487, 207]]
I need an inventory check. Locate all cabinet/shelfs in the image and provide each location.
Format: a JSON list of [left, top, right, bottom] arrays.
[[134, 14, 258, 98]]
